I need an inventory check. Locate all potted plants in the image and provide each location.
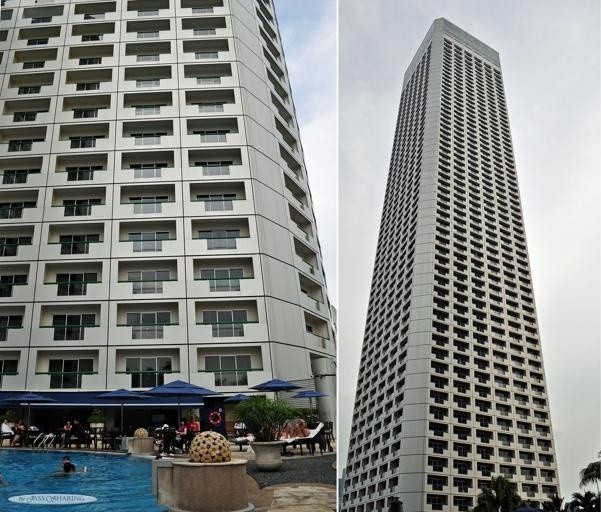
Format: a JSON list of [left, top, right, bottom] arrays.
[[88, 414, 106, 433], [231, 393, 304, 471]]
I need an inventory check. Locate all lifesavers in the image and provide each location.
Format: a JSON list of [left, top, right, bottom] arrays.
[[209, 411, 222, 426]]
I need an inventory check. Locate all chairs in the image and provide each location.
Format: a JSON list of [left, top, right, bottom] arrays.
[[231, 422, 334, 456], [0, 426, 196, 458]]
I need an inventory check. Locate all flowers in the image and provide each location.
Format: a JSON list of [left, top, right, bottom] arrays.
[[190, 431, 231, 463]]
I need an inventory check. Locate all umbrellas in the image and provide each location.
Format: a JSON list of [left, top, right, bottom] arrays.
[[137, 379, 224, 428], [223, 393, 250, 405], [92, 389, 151, 433], [247, 378, 304, 400], [289, 390, 328, 416], [0, 393, 57, 427]]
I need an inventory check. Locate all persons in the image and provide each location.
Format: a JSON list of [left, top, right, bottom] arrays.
[[62, 418, 82, 449], [13, 418, 28, 448], [55, 462, 72, 476], [69, 464, 75, 473], [1, 418, 20, 448], [62, 456, 70, 464], [60, 421, 72, 448], [283, 418, 311, 439], [166, 418, 188, 456]]
